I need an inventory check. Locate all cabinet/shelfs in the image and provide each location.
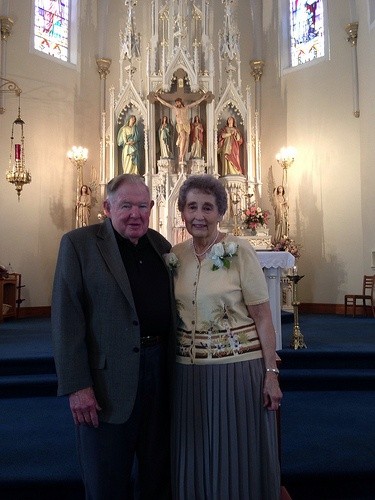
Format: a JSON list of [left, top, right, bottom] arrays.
[[0, 273, 18, 322]]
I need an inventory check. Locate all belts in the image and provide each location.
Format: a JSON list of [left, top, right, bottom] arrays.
[[140, 335, 168, 347]]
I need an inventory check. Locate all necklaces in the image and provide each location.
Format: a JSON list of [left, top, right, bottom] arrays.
[[192, 231, 219, 257]]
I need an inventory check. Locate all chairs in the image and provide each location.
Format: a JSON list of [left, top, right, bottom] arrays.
[[344, 275, 375, 319]]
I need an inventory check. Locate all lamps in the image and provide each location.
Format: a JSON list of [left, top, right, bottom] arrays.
[[66, 146, 90, 169], [0, 77, 33, 202], [276, 146, 298, 169]]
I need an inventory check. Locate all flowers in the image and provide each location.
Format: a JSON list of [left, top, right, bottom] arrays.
[[208, 241, 239, 271], [164, 253, 179, 276], [242, 201, 271, 229]]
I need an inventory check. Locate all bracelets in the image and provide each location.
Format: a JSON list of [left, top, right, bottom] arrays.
[[266, 368, 279, 374]]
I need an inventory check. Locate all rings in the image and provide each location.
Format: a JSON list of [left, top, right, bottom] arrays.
[[279, 403, 281, 406]]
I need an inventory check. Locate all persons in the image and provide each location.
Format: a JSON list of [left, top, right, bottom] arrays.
[[154, 94, 209, 160], [52, 175, 178, 500], [217, 116, 243, 176], [273, 185, 289, 238], [190, 116, 204, 158], [117, 115, 141, 174], [77, 185, 91, 228], [159, 116, 173, 158], [170, 175, 283, 500]]
